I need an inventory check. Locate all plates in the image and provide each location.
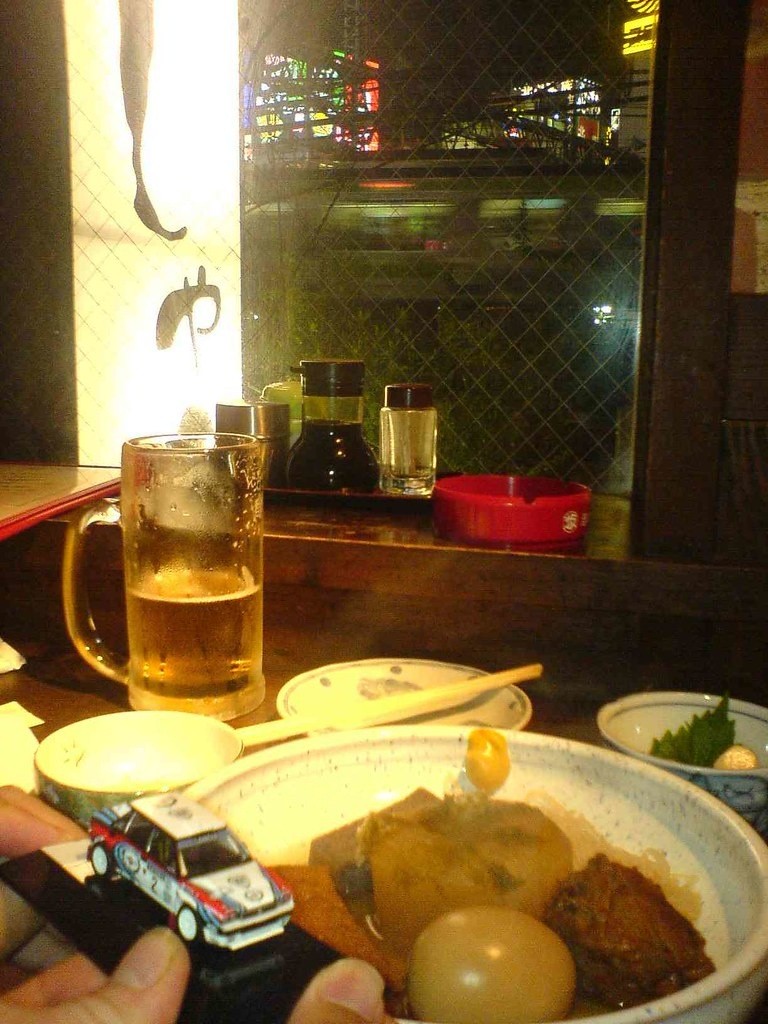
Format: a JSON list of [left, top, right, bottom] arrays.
[[277, 657, 532, 734]]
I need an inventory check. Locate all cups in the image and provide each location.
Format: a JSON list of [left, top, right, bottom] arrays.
[[62, 432, 267, 724], [216, 402, 290, 489]]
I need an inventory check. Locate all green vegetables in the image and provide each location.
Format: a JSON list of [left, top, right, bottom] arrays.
[[648, 693, 735, 768]]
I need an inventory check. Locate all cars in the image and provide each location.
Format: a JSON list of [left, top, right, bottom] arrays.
[[90, 790, 296, 950]]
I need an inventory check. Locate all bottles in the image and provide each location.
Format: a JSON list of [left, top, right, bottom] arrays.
[[283, 359, 379, 492], [378, 382, 437, 497], [259, 382, 302, 444]]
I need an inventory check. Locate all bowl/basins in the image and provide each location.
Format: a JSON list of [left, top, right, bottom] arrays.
[[597, 691, 767, 835], [32, 710, 243, 832], [182, 724, 768, 1023]]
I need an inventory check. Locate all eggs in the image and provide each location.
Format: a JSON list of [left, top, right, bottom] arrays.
[[407, 907, 575, 1024]]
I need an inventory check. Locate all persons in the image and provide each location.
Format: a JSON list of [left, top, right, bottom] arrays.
[[0, 781, 389, 1023]]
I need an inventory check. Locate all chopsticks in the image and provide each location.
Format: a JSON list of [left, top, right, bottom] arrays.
[[236, 661, 544, 745]]
